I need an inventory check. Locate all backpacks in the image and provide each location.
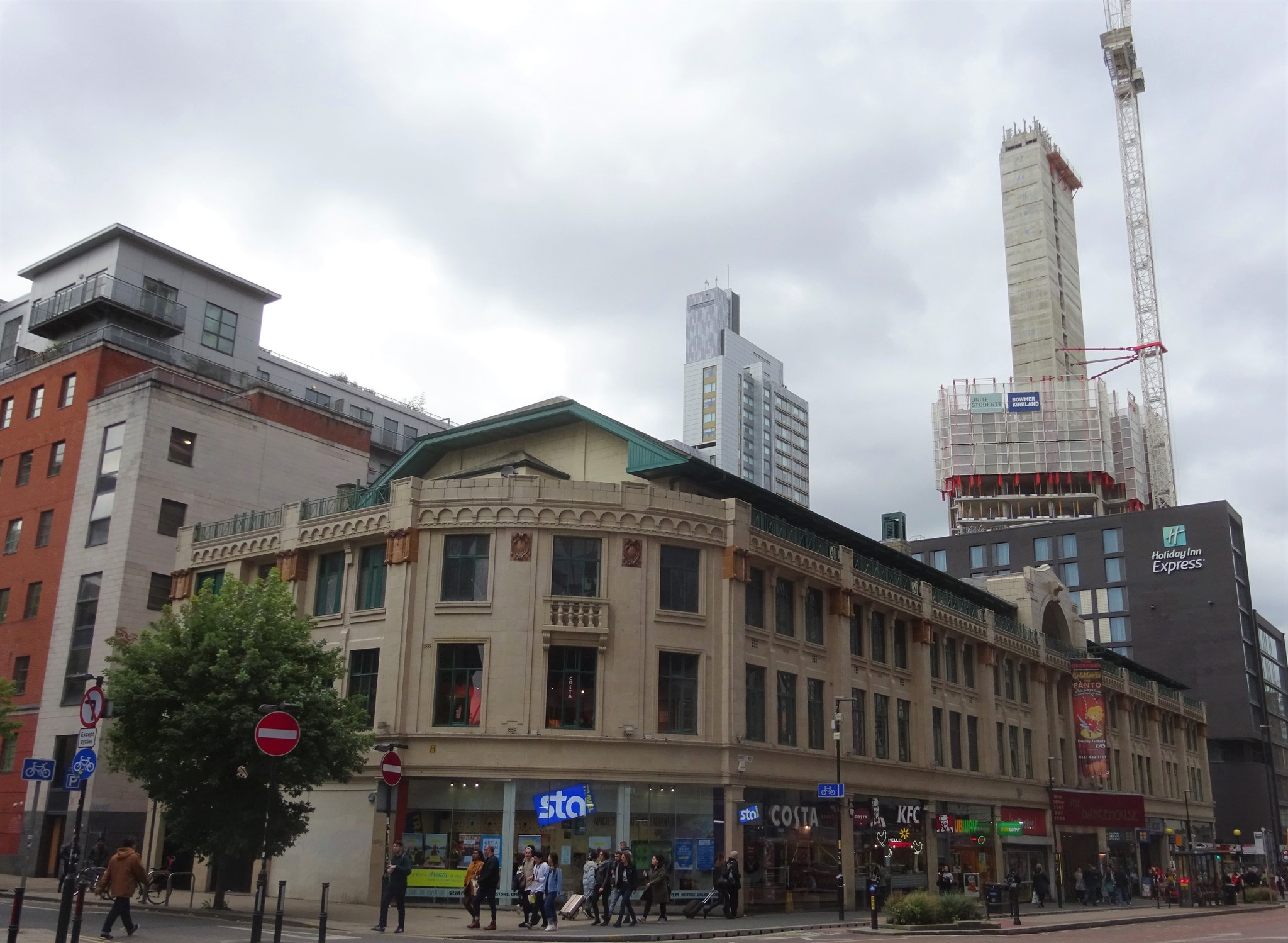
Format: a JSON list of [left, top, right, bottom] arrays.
[[1007, 873, 1016, 884]]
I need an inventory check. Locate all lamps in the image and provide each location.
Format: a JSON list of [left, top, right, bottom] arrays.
[[648, 784, 674, 791], [736, 733, 746, 740], [626, 727, 634, 734], [738, 761, 746, 772], [450, 782, 480, 787], [378, 721, 389, 729]]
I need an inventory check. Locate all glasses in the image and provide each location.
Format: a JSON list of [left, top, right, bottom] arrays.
[[484, 849, 488, 851]]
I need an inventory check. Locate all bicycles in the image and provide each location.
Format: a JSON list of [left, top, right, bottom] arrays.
[[105, 866, 174, 904], [73, 858, 118, 900]]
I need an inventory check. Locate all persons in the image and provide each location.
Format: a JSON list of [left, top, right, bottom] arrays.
[[722, 851, 741, 919], [591, 849, 612, 926], [637, 854, 672, 924], [1074, 862, 1132, 907], [402, 846, 418, 854], [948, 858, 964, 881], [94, 835, 151, 940], [544, 852, 563, 931], [450, 835, 465, 870], [370, 840, 413, 933], [869, 859, 888, 912], [938, 865, 954, 893], [744, 834, 759, 868], [1032, 863, 1050, 908], [574, 714, 592, 728], [87, 838, 108, 892], [462, 844, 500, 930], [57, 837, 80, 896], [431, 844, 440, 855], [582, 852, 599, 919], [798, 853, 810, 888], [516, 844, 549, 929], [1149, 866, 1179, 908], [703, 850, 731, 919], [1007, 867, 1021, 901], [1222, 866, 1287, 900], [602, 841, 639, 927]]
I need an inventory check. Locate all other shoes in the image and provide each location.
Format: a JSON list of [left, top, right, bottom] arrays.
[[591, 914, 638, 927], [467, 919, 497, 930], [732, 913, 741, 919], [727, 914, 736, 920], [518, 915, 559, 931], [703, 906, 711, 919], [516, 904, 523, 915]]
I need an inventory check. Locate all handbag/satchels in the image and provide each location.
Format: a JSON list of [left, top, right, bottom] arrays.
[[723, 866, 736, 885], [1031, 891, 1039, 904], [471, 875, 482, 896]]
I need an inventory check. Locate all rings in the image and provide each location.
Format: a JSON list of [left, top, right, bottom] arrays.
[[601, 890, 602, 891]]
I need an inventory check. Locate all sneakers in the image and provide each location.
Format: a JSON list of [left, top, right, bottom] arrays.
[[657, 918, 666, 924], [371, 926, 385, 932], [128, 924, 139, 935], [394, 927, 404, 933], [100, 934, 114, 940], [636, 915, 646, 923], [583, 908, 594, 919]]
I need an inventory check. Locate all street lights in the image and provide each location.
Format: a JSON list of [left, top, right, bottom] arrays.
[[1183, 789, 1197, 850], [835, 695, 859, 920]]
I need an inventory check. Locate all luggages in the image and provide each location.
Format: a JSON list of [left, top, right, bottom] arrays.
[[683, 892, 713, 919], [558, 890, 594, 921]]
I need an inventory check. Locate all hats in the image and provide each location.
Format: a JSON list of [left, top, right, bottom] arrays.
[[729, 850, 738, 860]]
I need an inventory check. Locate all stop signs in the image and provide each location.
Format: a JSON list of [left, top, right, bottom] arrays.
[[381, 750, 404, 786], [255, 711, 301, 756]]
